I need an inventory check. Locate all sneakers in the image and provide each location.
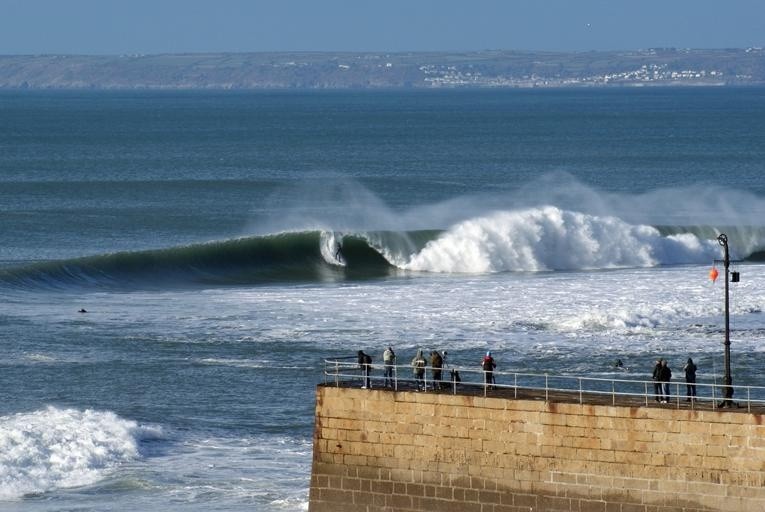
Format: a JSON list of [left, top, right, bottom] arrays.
[[361, 385, 442, 391], [655, 399, 669, 404], [693, 398, 696, 402], [684, 399, 690, 402]]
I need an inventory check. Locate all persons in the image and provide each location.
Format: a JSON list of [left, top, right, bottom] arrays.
[[382, 346, 396, 391], [429, 350, 443, 392], [357, 350, 373, 389], [656, 359, 672, 405], [652, 359, 663, 403], [682, 357, 697, 403], [480, 350, 497, 393], [410, 349, 428, 392]]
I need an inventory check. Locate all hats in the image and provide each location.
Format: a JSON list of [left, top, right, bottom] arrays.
[[656, 359, 661, 363], [661, 360, 667, 365]]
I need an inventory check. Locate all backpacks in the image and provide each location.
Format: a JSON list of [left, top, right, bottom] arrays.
[[364, 355, 372, 365], [416, 358, 426, 372]]
